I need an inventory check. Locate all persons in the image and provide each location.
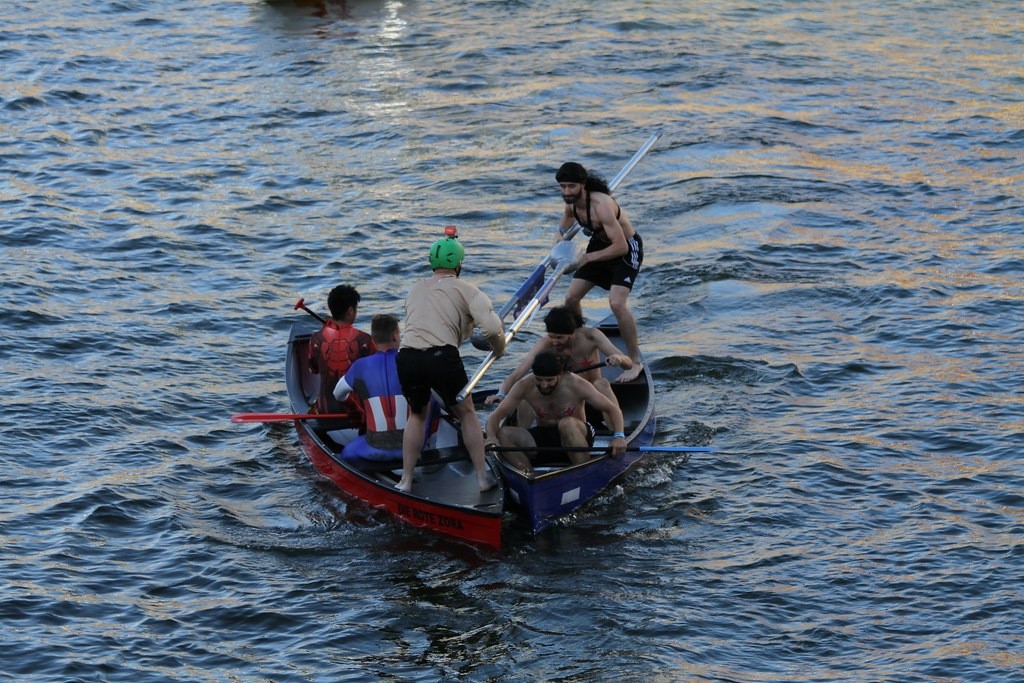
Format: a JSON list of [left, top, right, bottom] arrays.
[[484, 305, 633, 433], [307, 284, 376, 416], [484, 351, 628, 474], [395, 227, 506, 493], [556, 162, 644, 382], [332, 314, 440, 463]]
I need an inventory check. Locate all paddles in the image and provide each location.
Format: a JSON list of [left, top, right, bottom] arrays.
[[230, 413, 365, 421], [470, 358, 614, 396], [295, 297, 330, 326], [489, 445, 713, 454]]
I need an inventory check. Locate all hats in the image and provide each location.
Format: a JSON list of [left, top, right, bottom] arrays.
[[556, 162, 587, 183], [532, 352, 561, 377], [545, 309, 576, 335]]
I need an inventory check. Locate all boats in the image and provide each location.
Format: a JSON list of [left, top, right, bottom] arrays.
[[286, 314, 504, 549], [490, 314, 656, 534]]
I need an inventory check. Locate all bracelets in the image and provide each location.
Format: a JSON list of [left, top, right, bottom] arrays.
[[496, 394, 505, 398], [557, 227, 566, 235], [613, 432, 625, 438]]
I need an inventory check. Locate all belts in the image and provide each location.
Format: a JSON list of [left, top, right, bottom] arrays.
[[400, 344, 455, 353]]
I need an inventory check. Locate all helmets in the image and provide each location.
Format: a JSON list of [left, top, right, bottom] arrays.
[[428, 238, 465, 273]]
